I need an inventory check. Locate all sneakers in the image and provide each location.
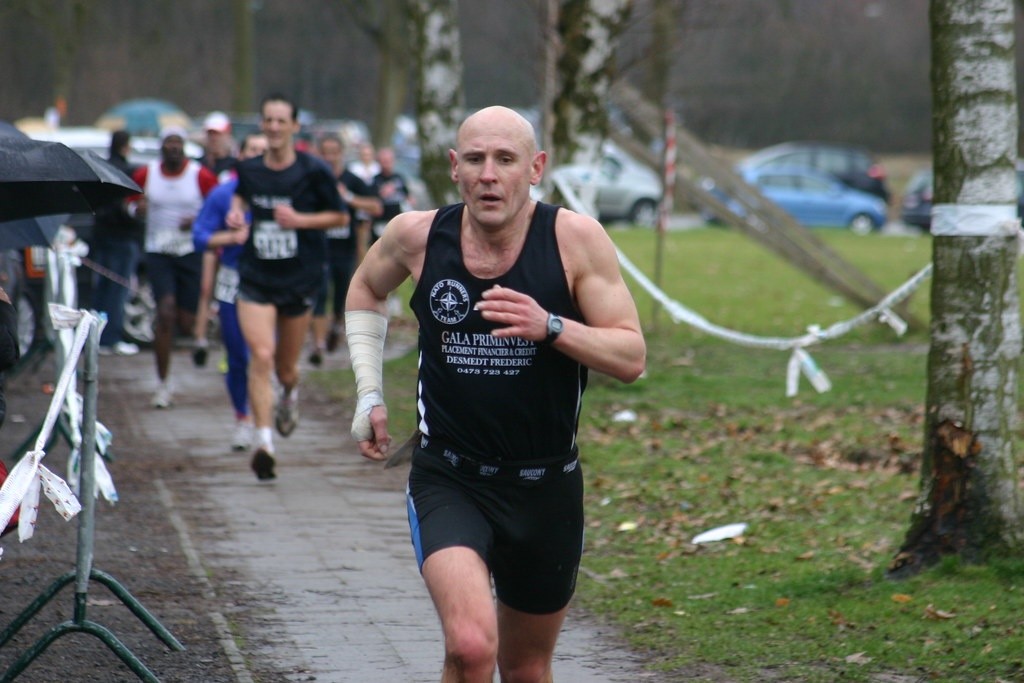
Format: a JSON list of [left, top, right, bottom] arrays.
[[276, 385, 300, 437], [251, 449, 276, 480]]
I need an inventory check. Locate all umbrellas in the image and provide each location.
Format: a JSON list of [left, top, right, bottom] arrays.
[[0, 213, 74, 250], [0, 120, 143, 224]]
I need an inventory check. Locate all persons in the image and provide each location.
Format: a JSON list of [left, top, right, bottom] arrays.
[[92, 92, 419, 482], [0, 287, 20, 560], [343, 106, 649, 683]]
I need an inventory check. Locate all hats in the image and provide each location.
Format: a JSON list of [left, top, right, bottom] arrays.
[[203, 111, 230, 133]]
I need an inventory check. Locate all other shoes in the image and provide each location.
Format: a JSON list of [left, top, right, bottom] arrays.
[[328, 332, 338, 352], [311, 350, 322, 365], [193, 349, 207, 368], [150, 380, 173, 409], [231, 417, 251, 450], [97, 342, 139, 356]]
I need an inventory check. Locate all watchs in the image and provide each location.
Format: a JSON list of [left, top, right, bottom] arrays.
[[541, 311, 564, 346]]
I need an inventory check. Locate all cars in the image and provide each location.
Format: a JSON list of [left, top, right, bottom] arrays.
[[533, 135, 666, 234], [691, 167, 892, 236], [743, 144, 896, 203], [903, 157, 1024, 231], [0, 243, 43, 369]]
